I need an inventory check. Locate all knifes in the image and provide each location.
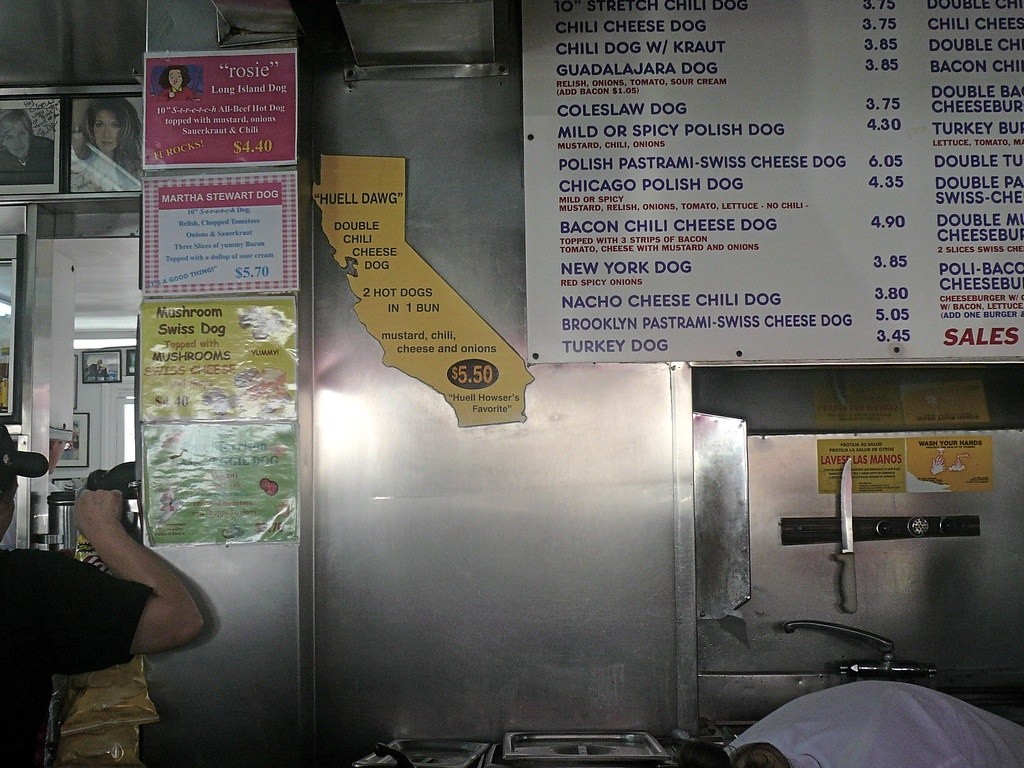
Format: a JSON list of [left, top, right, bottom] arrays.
[[836, 458, 857, 614]]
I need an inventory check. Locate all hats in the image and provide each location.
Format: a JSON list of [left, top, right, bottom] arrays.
[[0, 423, 49, 478]]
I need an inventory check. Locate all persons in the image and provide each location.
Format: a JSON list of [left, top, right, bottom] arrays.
[[0, 109, 54, 185], [0, 422, 204, 768], [85, 359, 108, 380], [73, 98, 143, 191], [49, 439, 68, 492], [680, 680, 1024, 768]]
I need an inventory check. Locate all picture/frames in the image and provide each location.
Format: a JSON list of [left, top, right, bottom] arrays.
[[81, 350, 122, 384], [56, 413, 90, 468], [63, 91, 143, 193], [0, 260, 25, 424], [0, 92, 66, 197], [125, 349, 136, 375], [73, 355, 78, 409]]
